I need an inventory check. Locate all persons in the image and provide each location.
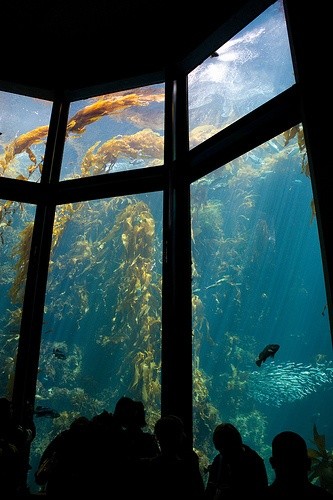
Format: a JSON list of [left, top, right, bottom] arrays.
[[0, 397, 32, 500], [268, 431, 333, 500], [206, 423, 268, 500], [34, 397, 207, 500]]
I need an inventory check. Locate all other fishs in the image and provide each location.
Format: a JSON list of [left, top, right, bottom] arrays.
[[255, 344, 280, 367], [34, 406, 53, 417], [52, 348, 66, 358]]
